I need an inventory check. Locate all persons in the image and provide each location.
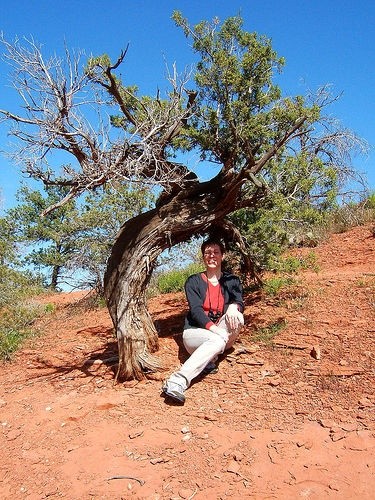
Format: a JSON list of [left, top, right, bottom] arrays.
[[162, 239, 245, 403]]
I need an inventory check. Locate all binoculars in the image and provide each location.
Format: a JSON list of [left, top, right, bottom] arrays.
[[208, 309, 221, 323]]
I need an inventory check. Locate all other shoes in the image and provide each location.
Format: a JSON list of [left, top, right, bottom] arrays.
[[206, 355, 218, 369], [163, 380, 186, 403]]
